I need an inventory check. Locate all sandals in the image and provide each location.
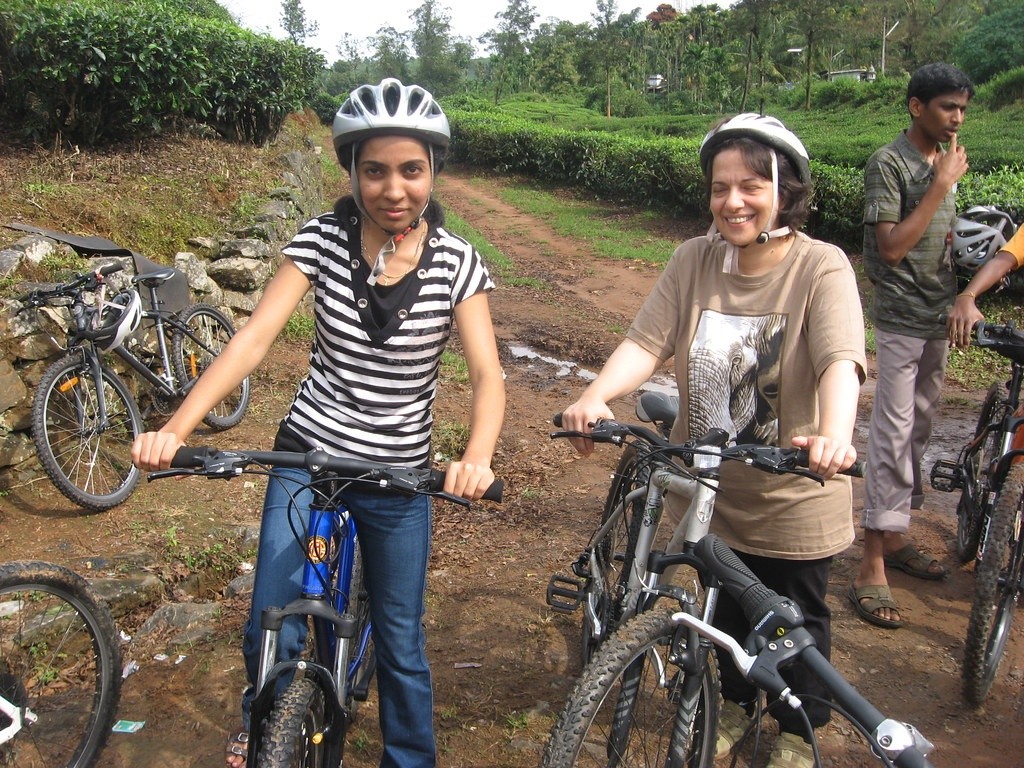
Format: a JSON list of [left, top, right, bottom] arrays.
[[224, 727, 249, 768]]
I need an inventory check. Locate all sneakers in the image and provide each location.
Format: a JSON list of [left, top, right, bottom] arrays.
[[765, 731, 815, 768], [713, 700, 761, 760]]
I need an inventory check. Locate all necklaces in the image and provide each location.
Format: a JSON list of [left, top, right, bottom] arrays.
[[361, 216, 427, 285]]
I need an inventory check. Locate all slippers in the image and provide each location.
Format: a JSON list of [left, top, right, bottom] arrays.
[[846, 571, 904, 632], [883, 543, 946, 580]]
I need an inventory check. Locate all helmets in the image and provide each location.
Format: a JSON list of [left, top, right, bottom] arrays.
[[699, 112, 812, 190], [330, 78, 451, 171], [952, 203, 1019, 274]]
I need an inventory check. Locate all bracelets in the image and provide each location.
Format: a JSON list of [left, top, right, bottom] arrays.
[[956, 291, 976, 300]]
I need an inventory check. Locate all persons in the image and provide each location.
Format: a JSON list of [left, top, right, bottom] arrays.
[[945, 222, 1024, 589], [847, 62, 1011, 627], [131, 77, 506, 768], [562, 111, 868, 768]]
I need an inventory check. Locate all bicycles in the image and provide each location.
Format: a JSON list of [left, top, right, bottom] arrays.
[[148, 438, 504, 768], [929, 313, 1024, 703], [0, 559, 124, 767], [671, 533, 937, 767], [539, 390, 869, 767], [13, 260, 249, 511]]
